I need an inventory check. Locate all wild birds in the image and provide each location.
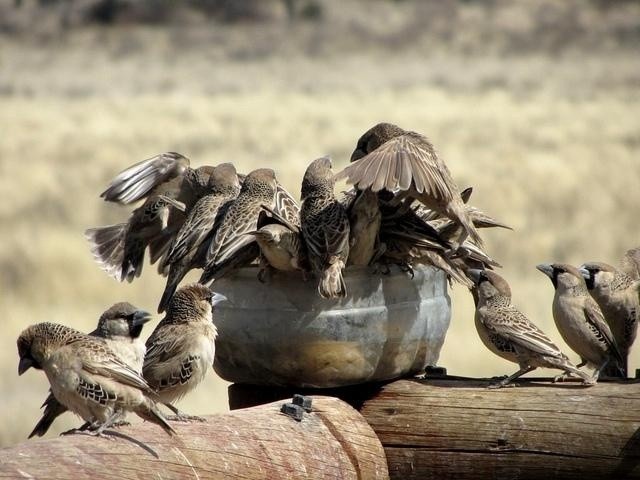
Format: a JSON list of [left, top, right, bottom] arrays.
[[464, 267, 593, 390], [579, 246, 640, 370], [16, 321, 178, 439], [83, 151, 513, 311], [28, 301, 153, 439], [143, 283, 228, 424], [535, 262, 627, 388], [331, 124, 486, 250]]
